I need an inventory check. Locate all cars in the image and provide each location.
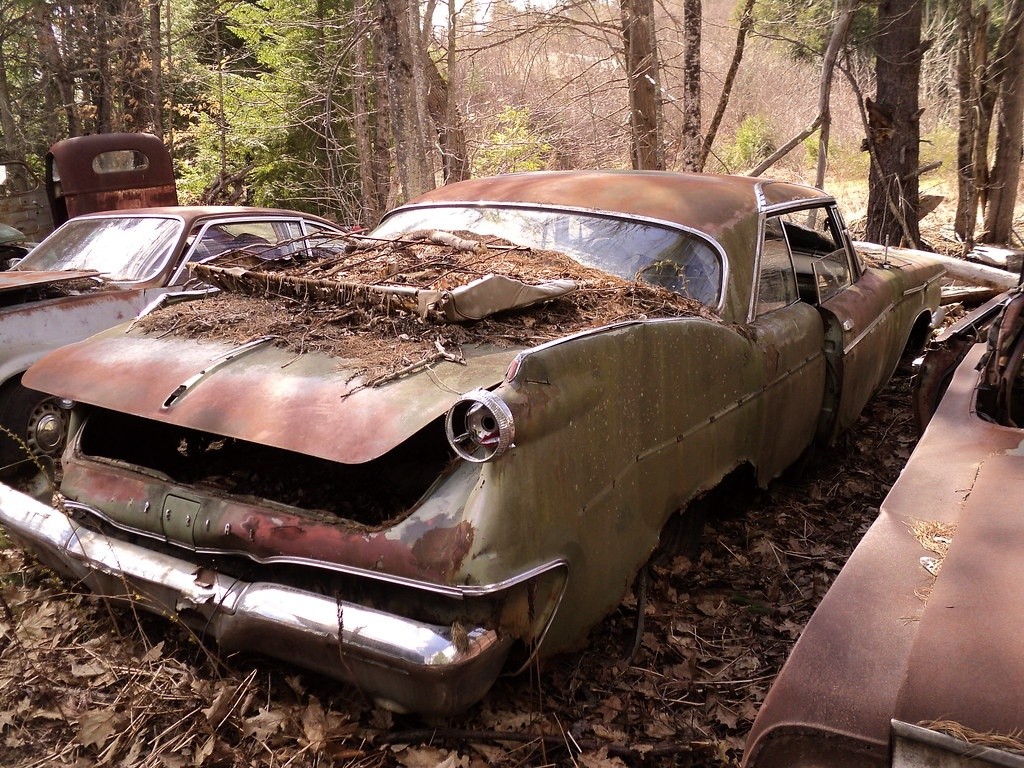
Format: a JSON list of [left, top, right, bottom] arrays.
[[0, 203, 354, 493], [735, 258, 1024, 768], [2, 171, 949, 724]]
